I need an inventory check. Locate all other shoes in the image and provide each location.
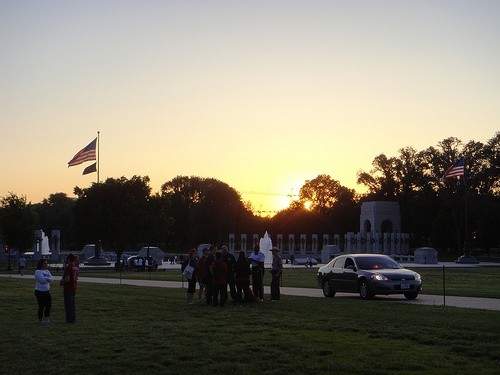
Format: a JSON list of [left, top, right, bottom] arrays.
[[40, 321, 45, 323], [189, 301, 195, 304], [44, 318, 51, 323], [271, 299, 278, 301], [260, 299, 264, 301]]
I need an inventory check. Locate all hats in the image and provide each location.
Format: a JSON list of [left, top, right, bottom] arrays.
[[269, 247, 281, 252]]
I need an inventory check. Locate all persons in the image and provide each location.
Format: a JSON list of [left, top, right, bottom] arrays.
[[267, 247, 283, 302], [305, 256, 312, 269], [18, 256, 27, 272], [186, 248, 198, 304], [235, 251, 250, 294], [60, 253, 79, 328], [179, 253, 183, 264], [248, 244, 265, 302], [34, 259, 54, 324], [197, 245, 237, 310], [171, 255, 178, 264], [288, 254, 295, 264]]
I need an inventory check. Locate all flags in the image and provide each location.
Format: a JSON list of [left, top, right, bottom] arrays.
[[443, 159, 464, 178], [68, 137, 96, 168], [83, 162, 96, 174]]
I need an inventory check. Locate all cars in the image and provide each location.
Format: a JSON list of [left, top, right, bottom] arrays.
[[316, 254, 423, 300]]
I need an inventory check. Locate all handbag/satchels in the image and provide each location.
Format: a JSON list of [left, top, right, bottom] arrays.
[[182, 258, 195, 280]]
